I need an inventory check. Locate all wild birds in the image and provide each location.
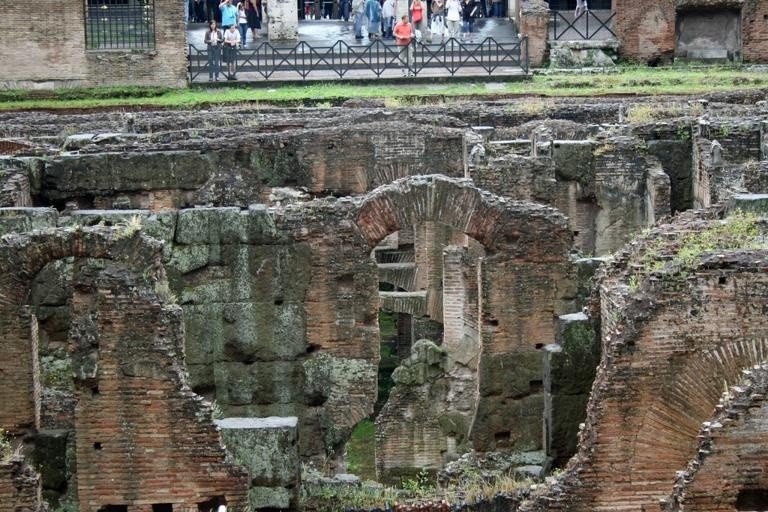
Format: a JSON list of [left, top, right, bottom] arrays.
[[553, 306, 588, 321], [535, 342, 562, 353]]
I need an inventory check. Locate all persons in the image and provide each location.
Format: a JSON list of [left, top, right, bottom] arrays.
[[204, 20, 225, 83], [221, 24, 241, 81], [392, 14, 416, 78], [188, 0, 507, 48]]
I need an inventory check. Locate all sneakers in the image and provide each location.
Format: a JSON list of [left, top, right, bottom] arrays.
[[401, 71, 414, 77], [209, 77, 238, 81]]
